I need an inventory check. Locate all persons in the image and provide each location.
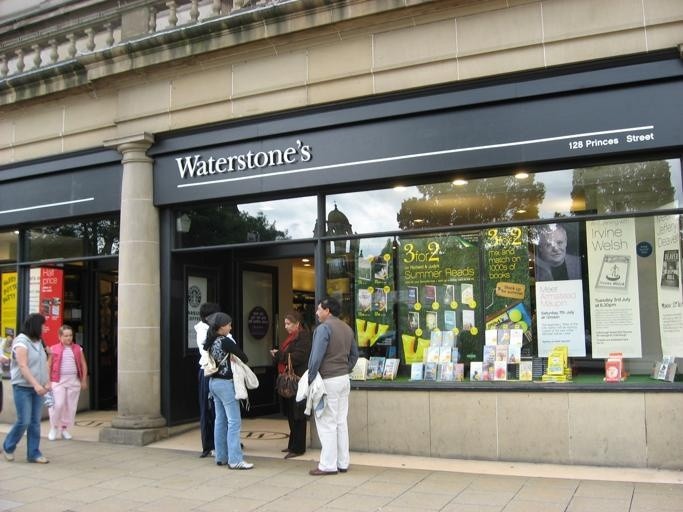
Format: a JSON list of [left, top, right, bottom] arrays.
[[193, 302, 237, 459], [306, 296, 359, 477], [45, 324, 88, 441], [201, 311, 254, 470], [0, 355, 10, 413], [533, 223, 580, 281], [267, 308, 311, 459], [1, 312, 52, 464]]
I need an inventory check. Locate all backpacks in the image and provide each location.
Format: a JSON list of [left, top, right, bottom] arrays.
[[198, 344, 229, 377]]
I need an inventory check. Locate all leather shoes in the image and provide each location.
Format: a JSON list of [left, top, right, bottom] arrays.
[[281, 448, 294, 452], [310, 468, 337, 475], [200, 449, 212, 458], [285, 452, 304, 458]]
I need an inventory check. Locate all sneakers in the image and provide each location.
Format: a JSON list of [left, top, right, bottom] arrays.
[[48, 428, 58, 441], [229, 461, 254, 469], [61, 430, 72, 439], [33, 456, 49, 463], [3, 448, 14, 462]]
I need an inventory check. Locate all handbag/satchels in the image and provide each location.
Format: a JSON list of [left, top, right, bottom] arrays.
[[274, 368, 301, 398]]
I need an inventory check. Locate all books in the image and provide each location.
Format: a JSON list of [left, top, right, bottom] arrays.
[[371, 255, 388, 280], [357, 256, 370, 281], [594, 255, 632, 291], [654, 355, 677, 382], [606, 352, 625, 381], [348, 287, 532, 347], [660, 250, 679, 290], [351, 357, 399, 380], [410, 328, 572, 381]]
